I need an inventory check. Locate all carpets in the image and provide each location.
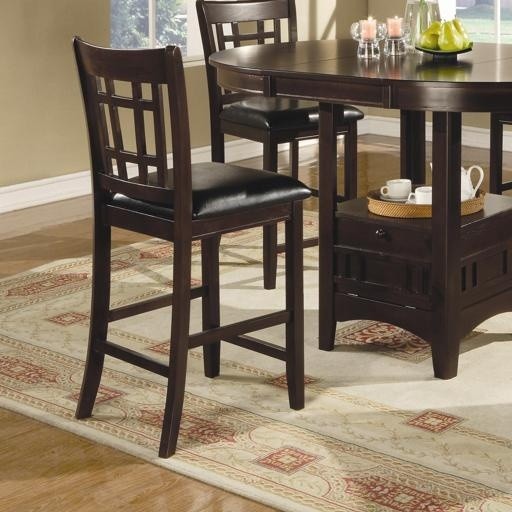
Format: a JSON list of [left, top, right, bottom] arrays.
[[1, 208, 511, 510]]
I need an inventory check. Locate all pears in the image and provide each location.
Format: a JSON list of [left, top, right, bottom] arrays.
[[419, 17, 470, 51]]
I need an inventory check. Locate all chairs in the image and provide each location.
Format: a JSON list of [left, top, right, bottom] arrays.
[[196, 0, 363, 290], [489, 110, 511, 197], [72, 36, 312, 458]]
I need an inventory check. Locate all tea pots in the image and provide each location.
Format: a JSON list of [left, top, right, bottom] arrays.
[[461, 162, 484, 203]]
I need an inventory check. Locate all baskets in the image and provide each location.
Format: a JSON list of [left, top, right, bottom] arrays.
[[367, 184, 485, 218]]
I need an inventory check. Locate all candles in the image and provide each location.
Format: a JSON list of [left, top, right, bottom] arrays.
[[360, 16, 377, 41], [387, 16, 404, 38]]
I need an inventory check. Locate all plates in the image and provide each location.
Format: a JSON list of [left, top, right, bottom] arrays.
[[416, 39, 474, 63]]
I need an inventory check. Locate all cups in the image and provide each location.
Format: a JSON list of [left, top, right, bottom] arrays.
[[409, 187, 433, 205], [381, 179, 412, 201]]
